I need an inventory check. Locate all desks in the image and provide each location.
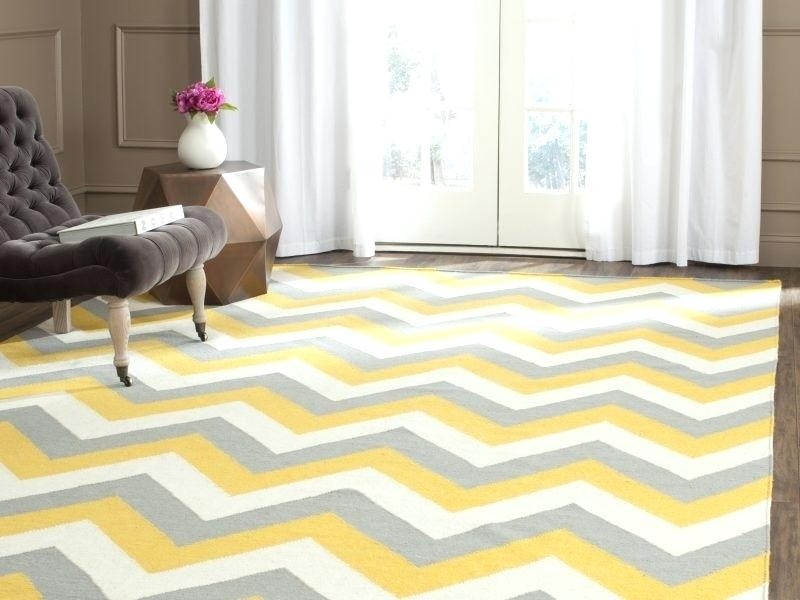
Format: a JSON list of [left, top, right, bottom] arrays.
[[133, 160, 284, 306]]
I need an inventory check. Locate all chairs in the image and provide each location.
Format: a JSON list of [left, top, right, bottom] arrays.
[[0, 86, 228, 387]]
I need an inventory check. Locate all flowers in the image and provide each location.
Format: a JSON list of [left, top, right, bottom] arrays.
[[170, 76, 240, 125]]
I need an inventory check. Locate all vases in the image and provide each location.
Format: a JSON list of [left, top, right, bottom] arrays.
[[178, 110, 228, 169]]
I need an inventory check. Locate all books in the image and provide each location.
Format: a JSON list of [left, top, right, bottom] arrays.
[[57, 204, 184, 244]]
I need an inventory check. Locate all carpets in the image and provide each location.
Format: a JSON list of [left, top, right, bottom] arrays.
[[0, 263, 781, 600]]
